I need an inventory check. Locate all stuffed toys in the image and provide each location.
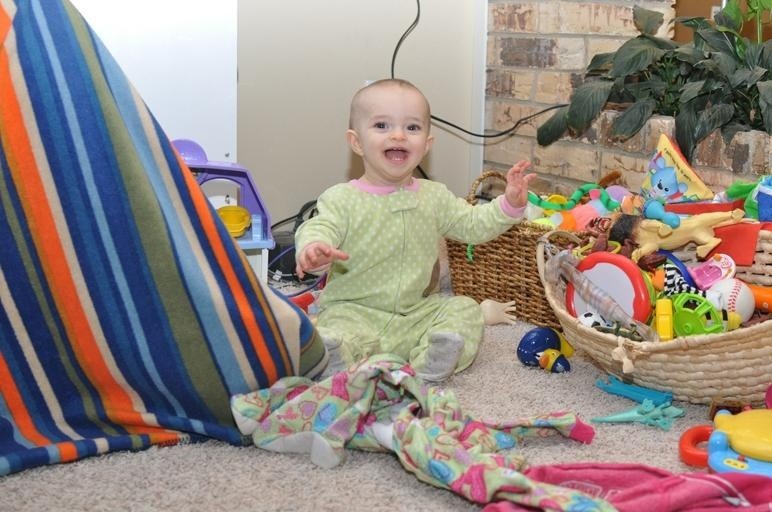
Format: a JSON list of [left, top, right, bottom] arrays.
[[517, 133, 772, 476]]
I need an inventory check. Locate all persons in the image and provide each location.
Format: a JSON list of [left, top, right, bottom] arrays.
[[294, 79, 538, 384]]
